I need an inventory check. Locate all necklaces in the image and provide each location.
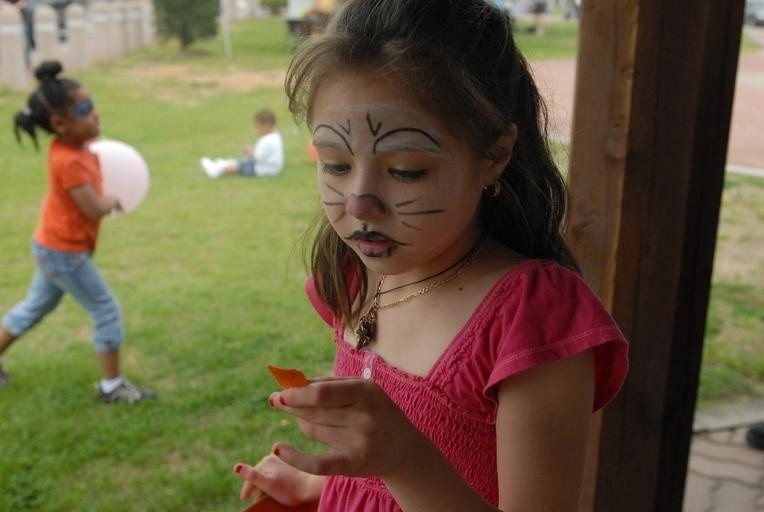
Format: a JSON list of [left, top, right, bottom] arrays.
[[355, 217, 489, 354]]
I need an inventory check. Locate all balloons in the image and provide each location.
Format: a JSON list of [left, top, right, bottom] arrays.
[[82, 137, 151, 217]]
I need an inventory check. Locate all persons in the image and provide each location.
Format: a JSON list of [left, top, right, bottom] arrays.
[[199, 104, 284, 181], [230, 1, 631, 511], [1, 61, 159, 403]]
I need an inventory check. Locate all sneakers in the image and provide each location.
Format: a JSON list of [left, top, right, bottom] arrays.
[[201, 156, 228, 180], [98, 379, 158, 405]]
